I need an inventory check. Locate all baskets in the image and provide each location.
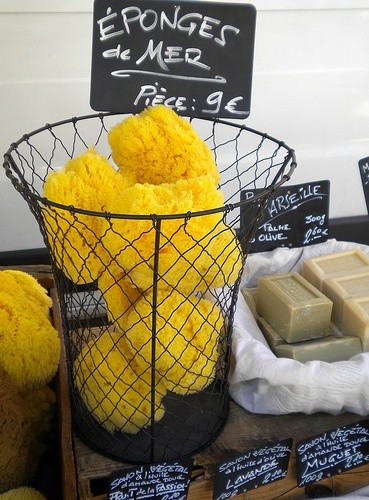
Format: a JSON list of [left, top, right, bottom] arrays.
[[4, 114, 297, 465]]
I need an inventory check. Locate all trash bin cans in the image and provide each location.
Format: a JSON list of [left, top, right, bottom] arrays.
[[5, 110, 296, 467]]
